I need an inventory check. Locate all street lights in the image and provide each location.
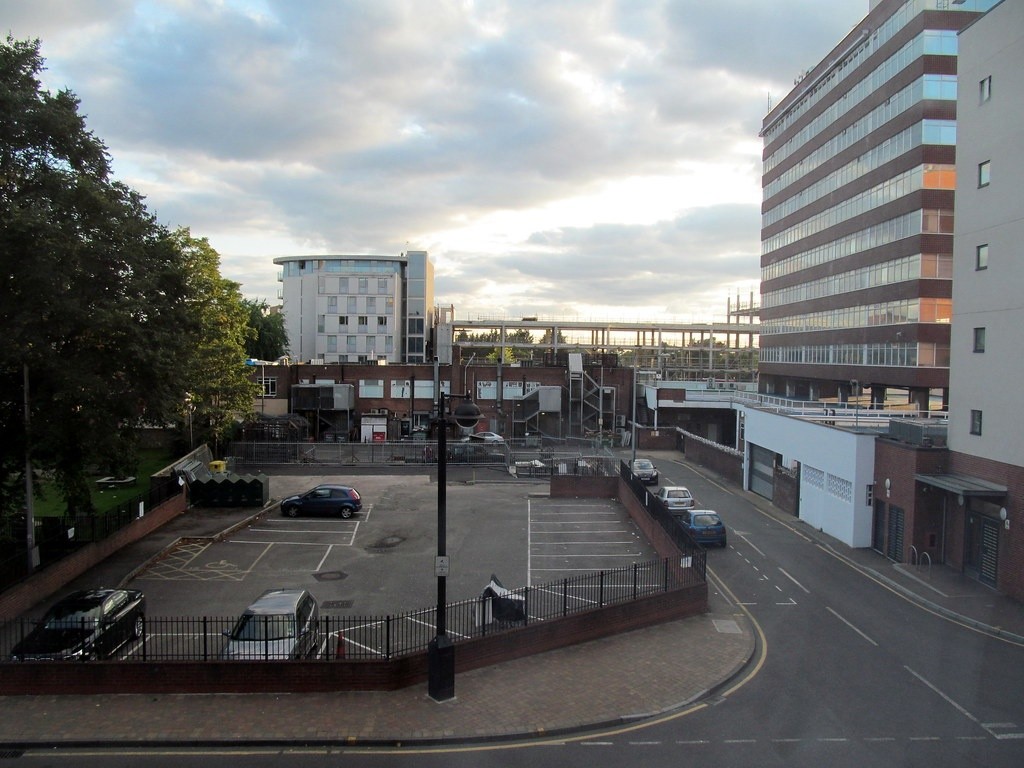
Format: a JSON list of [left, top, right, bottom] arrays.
[[592, 361, 603, 448], [425, 392, 486, 705]]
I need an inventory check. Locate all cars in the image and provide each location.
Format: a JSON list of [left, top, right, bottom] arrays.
[[461, 431, 505, 448], [654, 486, 695, 518], [628, 459, 659, 485], [280, 484, 362, 519], [8, 587, 146, 664], [680, 509, 727, 547], [217, 588, 319, 661]]
